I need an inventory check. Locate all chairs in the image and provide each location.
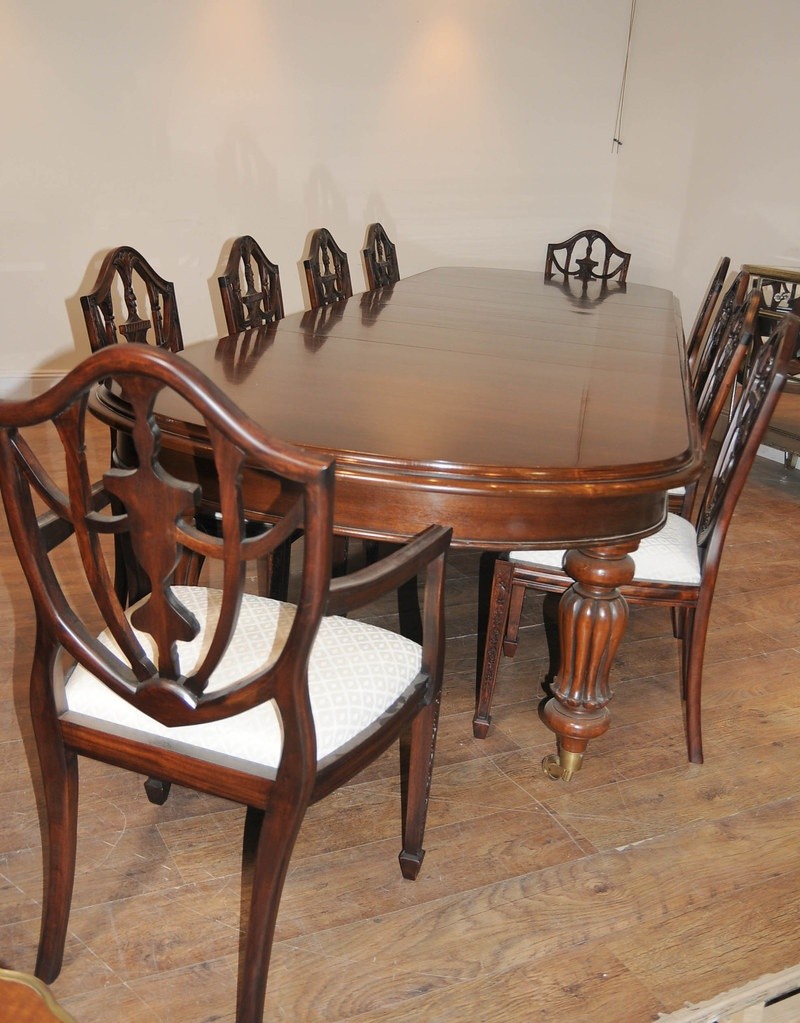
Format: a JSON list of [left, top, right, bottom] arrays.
[[362, 223, 400, 290], [81, 247, 346, 612], [0, 343, 450, 1023], [544, 229, 629, 283], [722, 264, 798, 456], [686, 255, 729, 359], [695, 272, 752, 393], [208, 235, 283, 338], [296, 228, 354, 311], [473, 317, 797, 763], [667, 289, 760, 510]]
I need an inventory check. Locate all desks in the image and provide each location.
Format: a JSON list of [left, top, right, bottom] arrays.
[[91, 265, 701, 782]]
